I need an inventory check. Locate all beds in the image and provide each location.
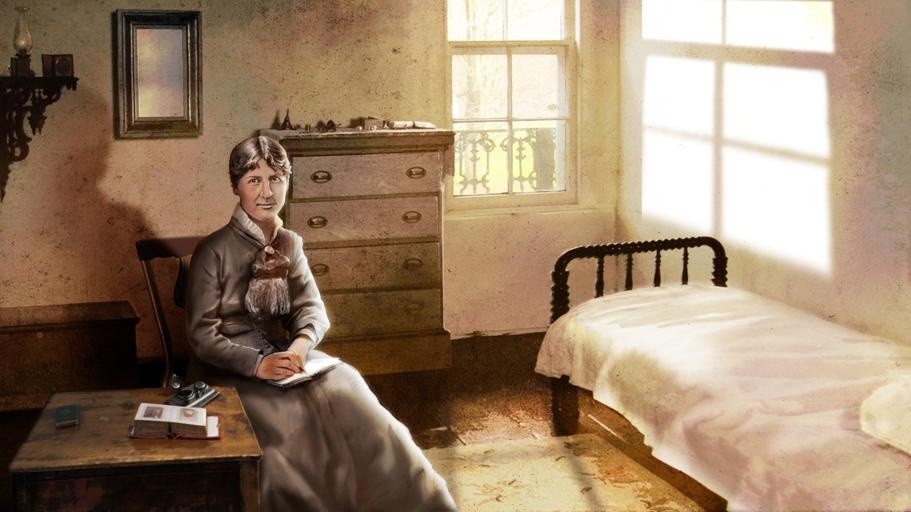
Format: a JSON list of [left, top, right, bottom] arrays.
[[533, 226, 911, 511]]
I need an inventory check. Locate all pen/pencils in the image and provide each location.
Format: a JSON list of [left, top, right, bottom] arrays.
[[262, 334, 307, 375]]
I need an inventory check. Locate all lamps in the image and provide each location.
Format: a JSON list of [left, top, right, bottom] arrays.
[[9, 6, 35, 77]]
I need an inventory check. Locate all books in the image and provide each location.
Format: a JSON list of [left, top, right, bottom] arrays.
[[260, 357, 344, 389]]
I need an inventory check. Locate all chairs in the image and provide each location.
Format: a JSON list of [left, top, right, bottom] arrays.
[[132, 236, 210, 388]]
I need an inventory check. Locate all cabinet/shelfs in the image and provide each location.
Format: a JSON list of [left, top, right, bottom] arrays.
[[259, 122, 454, 394]]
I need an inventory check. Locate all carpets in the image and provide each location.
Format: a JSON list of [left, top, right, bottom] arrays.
[[420, 433, 708, 512]]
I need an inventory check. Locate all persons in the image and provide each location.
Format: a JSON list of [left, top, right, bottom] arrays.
[[181, 137, 459, 510]]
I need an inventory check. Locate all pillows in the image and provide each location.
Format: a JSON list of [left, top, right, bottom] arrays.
[[858, 375, 911, 457]]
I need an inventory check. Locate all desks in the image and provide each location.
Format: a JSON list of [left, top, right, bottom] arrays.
[[11, 385, 266, 512]]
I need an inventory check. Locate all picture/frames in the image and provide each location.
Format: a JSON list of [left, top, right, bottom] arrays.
[[109, 7, 204, 139]]
